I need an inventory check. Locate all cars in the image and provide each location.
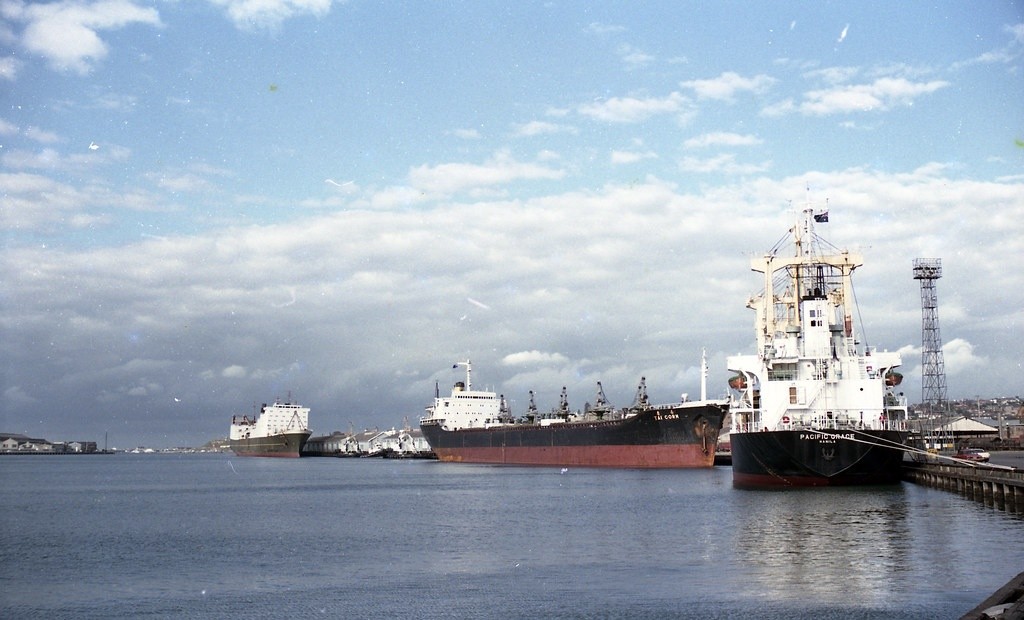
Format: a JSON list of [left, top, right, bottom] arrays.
[[952, 448, 990, 462]]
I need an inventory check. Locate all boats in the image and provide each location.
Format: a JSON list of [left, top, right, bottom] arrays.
[[727, 184, 910, 491], [419, 346, 730, 468], [886, 370, 903, 386], [229, 391, 313, 458], [728, 374, 747, 388]]
